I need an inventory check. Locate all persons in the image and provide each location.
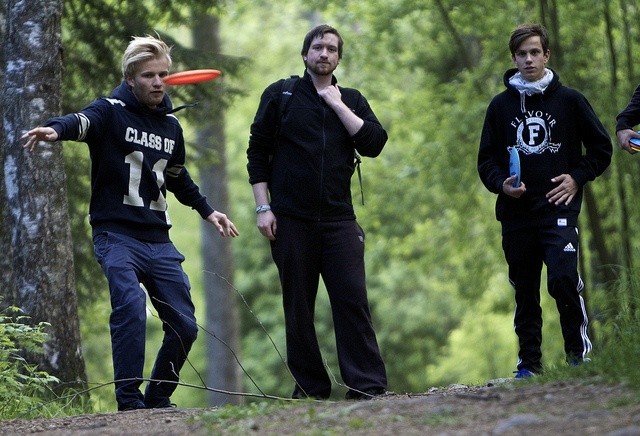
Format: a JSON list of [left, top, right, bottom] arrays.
[[245, 24, 388, 401], [21, 33, 239, 414], [614, 84, 640, 156], [475, 22, 614, 382]]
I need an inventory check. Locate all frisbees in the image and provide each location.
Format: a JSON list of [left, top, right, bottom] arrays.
[[509, 146, 521, 188], [162, 69, 221, 85], [629, 130, 640, 152]]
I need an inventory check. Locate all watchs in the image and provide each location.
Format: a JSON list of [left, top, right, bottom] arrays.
[[256, 206, 272, 214]]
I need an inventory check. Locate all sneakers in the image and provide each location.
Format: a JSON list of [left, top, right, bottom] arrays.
[[570, 358, 591, 366], [515, 369, 537, 379]]
